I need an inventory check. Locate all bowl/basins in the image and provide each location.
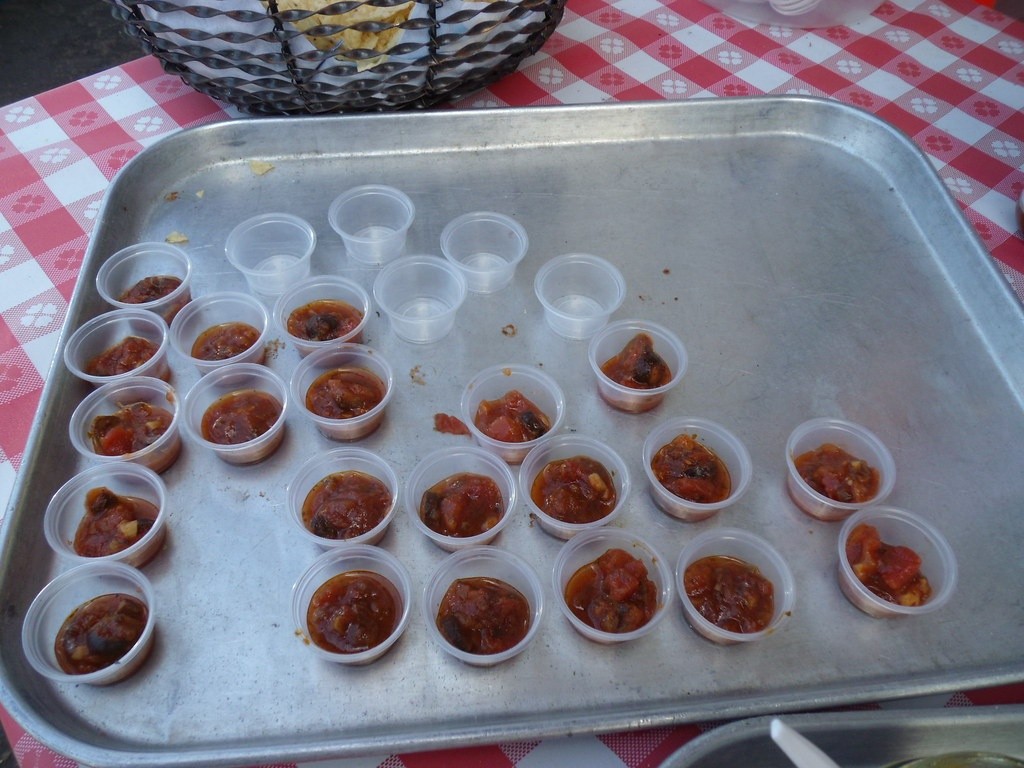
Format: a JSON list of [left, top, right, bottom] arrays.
[[95, 241, 193, 338], [64, 308, 172, 406], [69, 376, 181, 483], [21, 561, 157, 688], [289, 343, 396, 444], [181, 362, 289, 467], [640, 417, 754, 522], [224, 213, 318, 295], [327, 184, 529, 345], [284, 446, 415, 667], [784, 416, 959, 618], [168, 292, 271, 388], [271, 275, 371, 369], [675, 527, 799, 646], [44, 462, 169, 572], [404, 363, 674, 668], [532, 252, 689, 414]]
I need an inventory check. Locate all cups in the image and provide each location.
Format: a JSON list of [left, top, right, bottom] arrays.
[[878, 750, 1024, 768]]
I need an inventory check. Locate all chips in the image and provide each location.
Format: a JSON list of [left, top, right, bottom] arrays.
[[261, 0, 417, 72]]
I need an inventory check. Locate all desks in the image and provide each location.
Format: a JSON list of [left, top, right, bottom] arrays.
[[0, 0, 1024, 768]]
